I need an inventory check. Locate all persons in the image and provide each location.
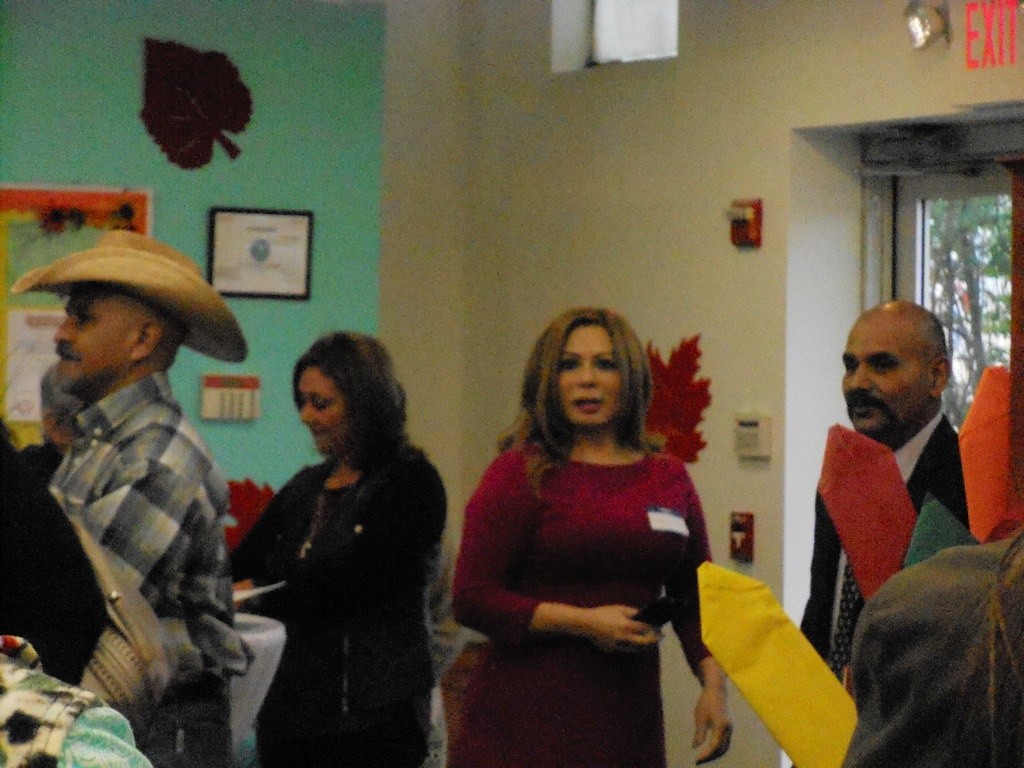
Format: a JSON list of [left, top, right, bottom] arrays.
[[799, 301, 1024, 768], [232, 332, 447, 768], [0, 230, 250, 768], [441, 307, 733, 768]]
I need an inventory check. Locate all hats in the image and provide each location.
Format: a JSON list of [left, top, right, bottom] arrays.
[[10, 229, 249, 363]]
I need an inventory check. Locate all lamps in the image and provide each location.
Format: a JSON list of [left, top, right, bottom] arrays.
[[902, 0, 1024, 108]]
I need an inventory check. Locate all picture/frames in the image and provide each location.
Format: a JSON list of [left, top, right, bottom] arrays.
[[208, 205, 314, 301]]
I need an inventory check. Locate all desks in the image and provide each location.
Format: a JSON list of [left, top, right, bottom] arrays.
[[230, 613, 287, 768]]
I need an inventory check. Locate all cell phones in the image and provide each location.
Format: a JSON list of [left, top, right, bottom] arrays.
[[631, 596, 685, 628]]
